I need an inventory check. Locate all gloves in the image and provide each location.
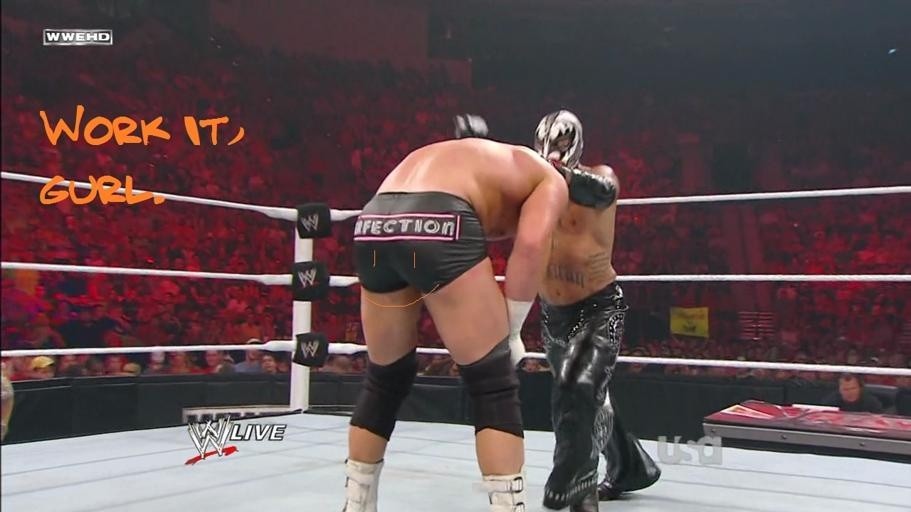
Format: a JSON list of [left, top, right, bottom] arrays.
[[454, 114, 489, 138]]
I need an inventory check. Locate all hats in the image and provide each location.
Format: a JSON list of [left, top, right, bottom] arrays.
[[30, 357, 55, 368]]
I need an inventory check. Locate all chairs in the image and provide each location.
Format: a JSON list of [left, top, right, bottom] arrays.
[[863, 383, 901, 416]]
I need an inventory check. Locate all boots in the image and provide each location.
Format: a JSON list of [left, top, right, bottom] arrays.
[[343, 459, 384, 512], [482, 465, 525, 512]]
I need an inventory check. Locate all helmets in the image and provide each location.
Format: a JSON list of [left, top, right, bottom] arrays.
[[534, 111, 585, 168]]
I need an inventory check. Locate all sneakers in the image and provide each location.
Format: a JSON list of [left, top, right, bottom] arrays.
[[571, 490, 598, 511], [598, 479, 617, 501]]
